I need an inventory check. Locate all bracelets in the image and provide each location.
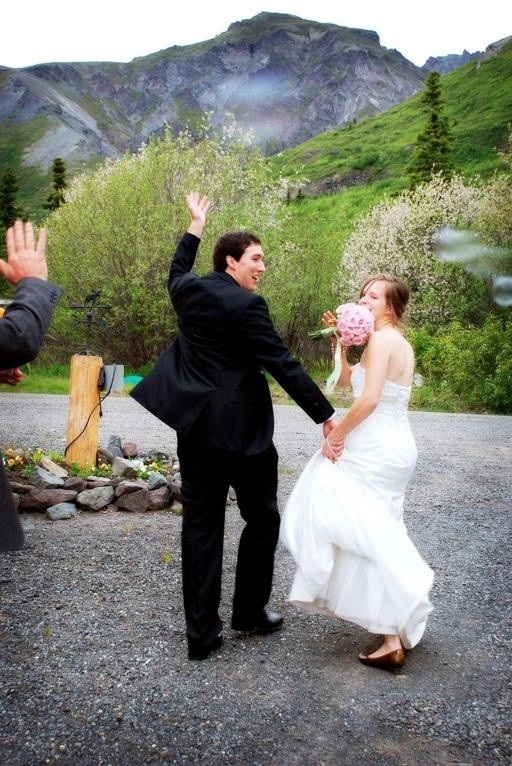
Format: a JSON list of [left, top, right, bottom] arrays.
[[326, 435, 338, 461]]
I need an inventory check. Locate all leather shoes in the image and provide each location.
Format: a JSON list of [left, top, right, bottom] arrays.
[[187, 631, 223, 662], [230, 609, 284, 634], [356, 646, 408, 670]]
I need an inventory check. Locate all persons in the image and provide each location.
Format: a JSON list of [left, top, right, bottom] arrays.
[[128, 189, 346, 662], [1, 218, 65, 553], [319, 273, 432, 671]]
